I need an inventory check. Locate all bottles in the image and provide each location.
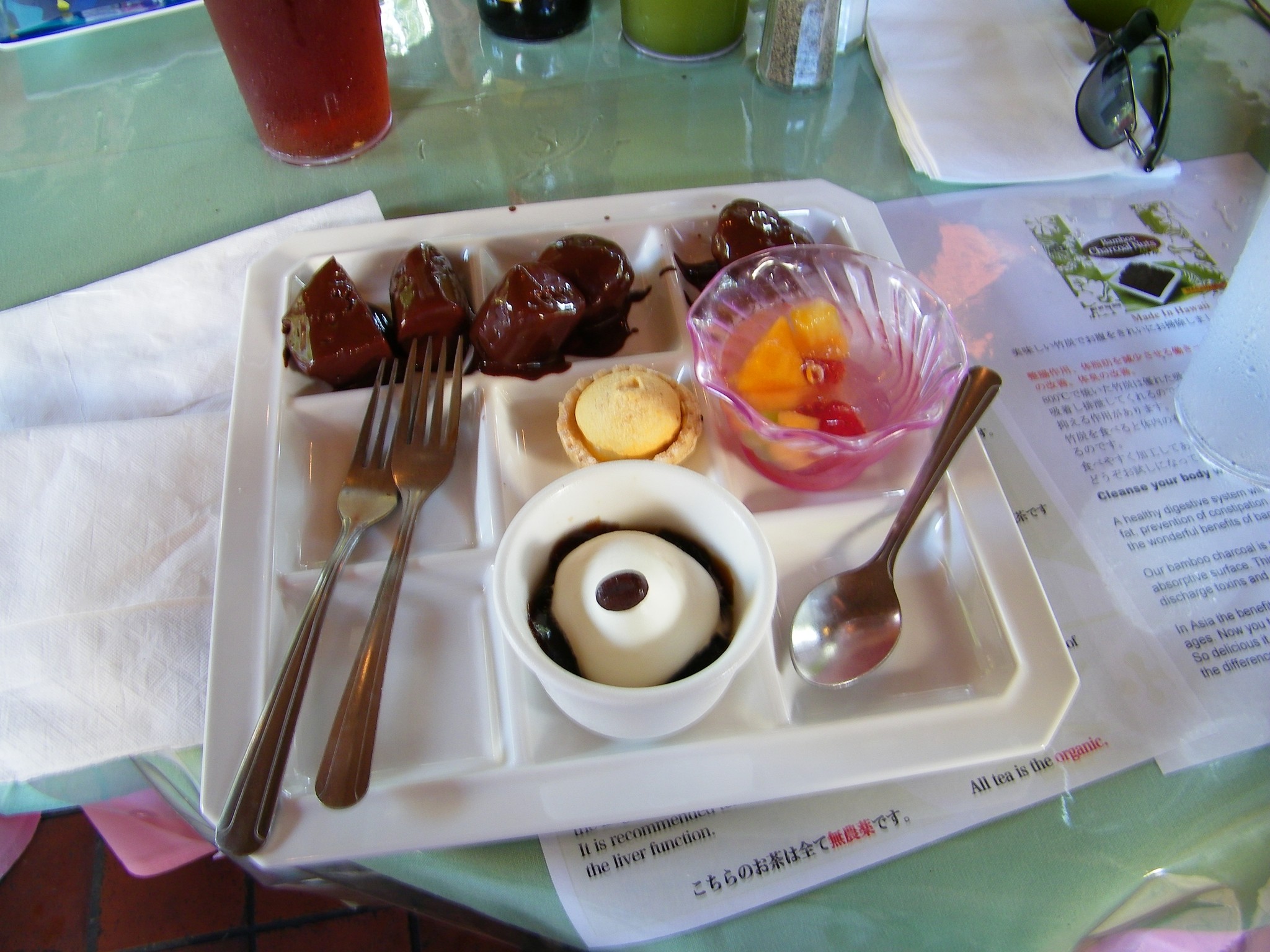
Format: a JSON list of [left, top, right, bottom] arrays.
[[476, 0, 596, 44], [835, 0, 868, 56], [756, 0, 841, 95]]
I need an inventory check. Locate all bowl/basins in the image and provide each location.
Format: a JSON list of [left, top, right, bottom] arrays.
[[685, 243, 970, 491]]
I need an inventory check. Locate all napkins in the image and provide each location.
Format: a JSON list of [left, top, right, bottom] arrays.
[[866, 0, 1182, 183], [0, 190, 381, 787]]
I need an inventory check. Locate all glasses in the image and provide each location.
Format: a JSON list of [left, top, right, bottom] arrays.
[[1075, 5, 1174, 172]]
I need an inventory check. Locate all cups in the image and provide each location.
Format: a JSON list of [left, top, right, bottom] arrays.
[[490, 460, 777, 739], [620, 0, 749, 61], [203, 0, 394, 165], [1171, 190, 1270, 489]]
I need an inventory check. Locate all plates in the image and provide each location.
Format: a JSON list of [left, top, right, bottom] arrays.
[[1108, 264, 1180, 303]]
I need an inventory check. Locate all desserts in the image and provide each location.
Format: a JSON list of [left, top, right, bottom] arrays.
[[528, 523, 733, 690], [555, 365, 705, 469]]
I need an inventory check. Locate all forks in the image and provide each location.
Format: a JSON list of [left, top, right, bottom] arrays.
[[313, 335, 462, 808], [214, 351, 418, 856]]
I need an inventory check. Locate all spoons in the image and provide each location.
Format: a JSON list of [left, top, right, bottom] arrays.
[[786, 362, 999, 687]]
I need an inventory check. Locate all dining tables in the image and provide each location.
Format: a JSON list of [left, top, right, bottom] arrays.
[[0, 0, 1270, 952]]
